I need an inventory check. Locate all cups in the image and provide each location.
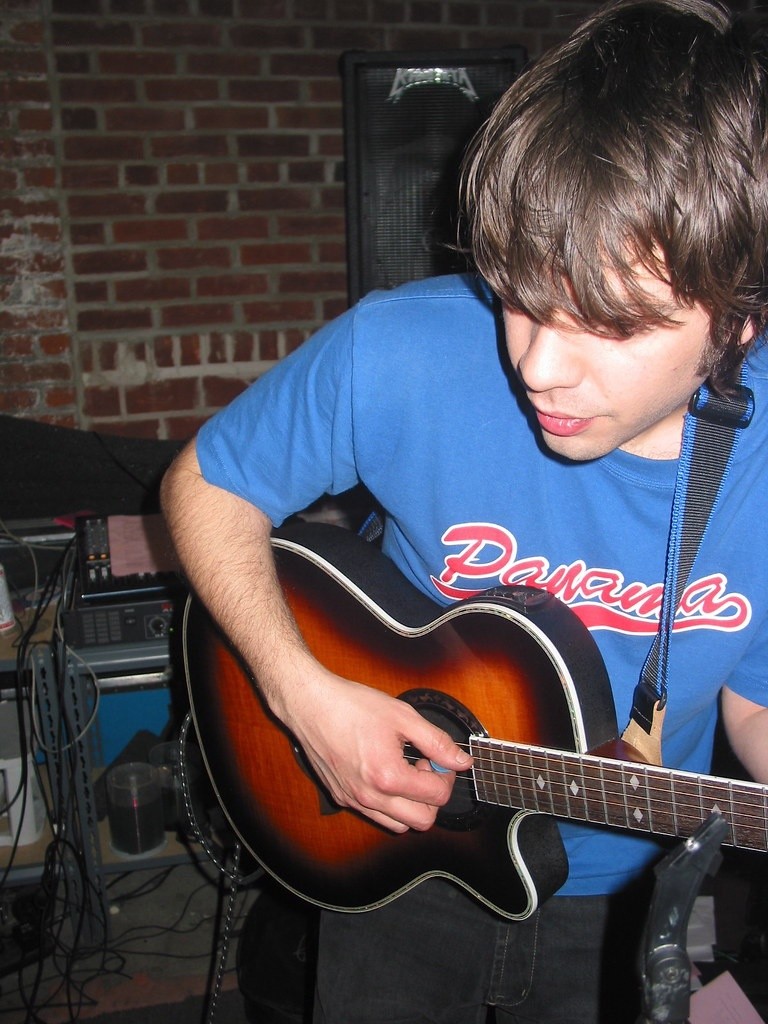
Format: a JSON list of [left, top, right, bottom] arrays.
[[149, 739, 202, 825], [108, 762, 166, 855]]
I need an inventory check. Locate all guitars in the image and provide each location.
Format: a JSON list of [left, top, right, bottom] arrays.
[[170, 518, 768, 926]]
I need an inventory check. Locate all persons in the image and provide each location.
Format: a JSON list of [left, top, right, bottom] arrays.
[[161, 0, 767, 1024]]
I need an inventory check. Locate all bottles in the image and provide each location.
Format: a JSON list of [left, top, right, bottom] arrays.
[[0, 565, 17, 639]]
